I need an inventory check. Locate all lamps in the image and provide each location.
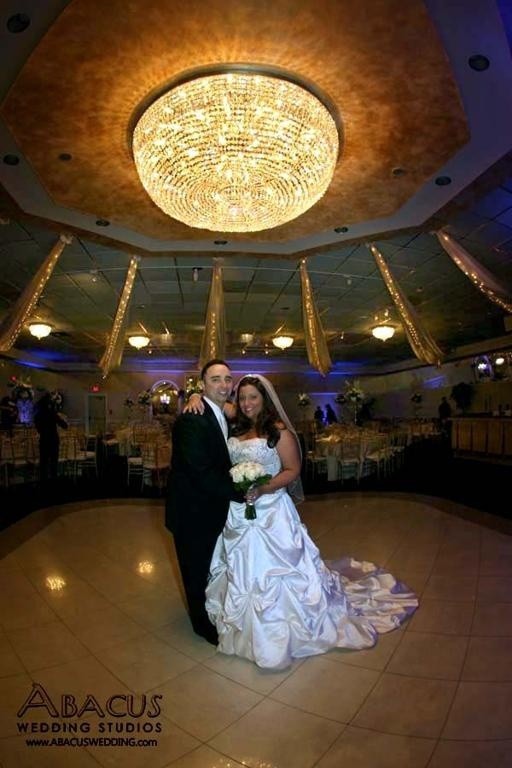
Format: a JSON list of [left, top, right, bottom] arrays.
[[27, 322, 52, 342], [124, 64, 344, 238], [371, 323, 396, 343], [126, 334, 152, 352], [270, 333, 294, 353]]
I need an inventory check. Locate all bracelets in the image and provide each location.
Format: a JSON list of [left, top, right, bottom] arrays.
[[189, 393, 201, 399]]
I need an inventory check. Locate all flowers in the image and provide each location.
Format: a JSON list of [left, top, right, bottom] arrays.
[[228, 460, 273, 520], [121, 385, 203, 414], [4, 373, 36, 403], [334, 375, 366, 412]]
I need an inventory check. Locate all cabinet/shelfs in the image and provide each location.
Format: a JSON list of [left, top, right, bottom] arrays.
[[445, 416, 511, 467]]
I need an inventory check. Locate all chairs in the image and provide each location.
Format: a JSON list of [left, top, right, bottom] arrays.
[[0, 418, 442, 494]]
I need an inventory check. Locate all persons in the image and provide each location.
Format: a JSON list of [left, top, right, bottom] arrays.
[[31, 389, 69, 492], [324, 403, 338, 424], [438, 396, 452, 418], [163, 358, 286, 646], [356, 402, 373, 427], [314, 406, 324, 422], [182, 373, 422, 672]]
